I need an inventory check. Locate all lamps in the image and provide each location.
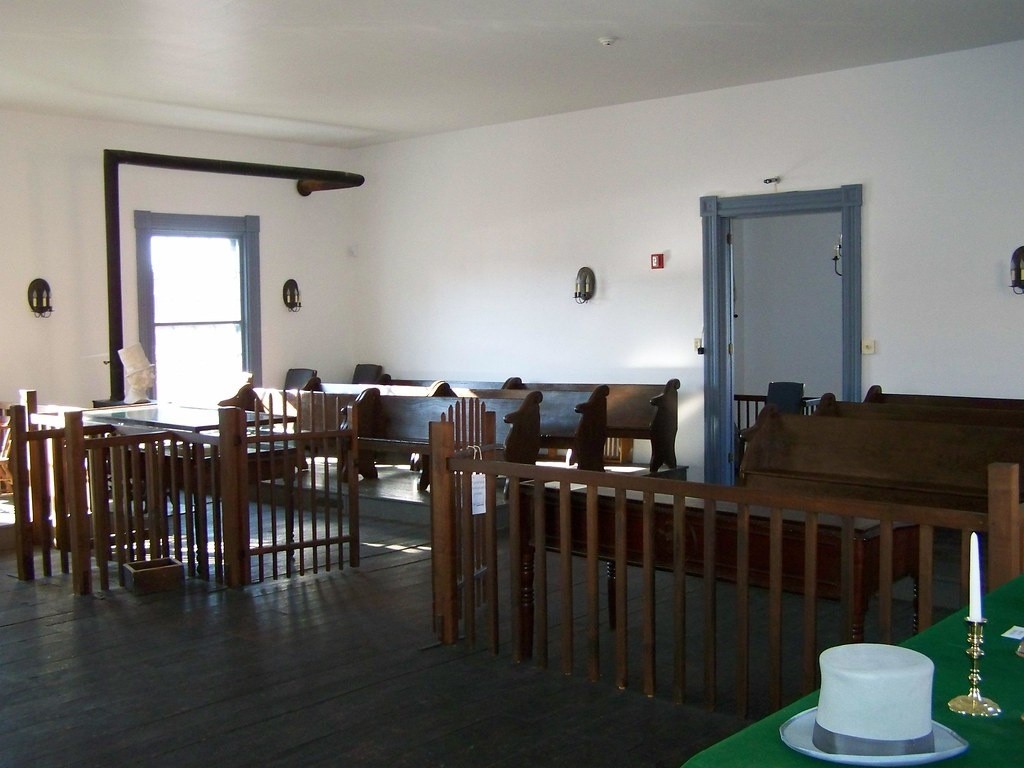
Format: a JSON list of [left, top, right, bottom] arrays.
[[283, 279, 302, 313], [27, 278, 55, 318], [1009, 246, 1024, 294], [573, 267, 595, 304]]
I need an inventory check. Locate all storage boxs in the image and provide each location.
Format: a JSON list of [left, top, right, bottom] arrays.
[[122, 557, 186, 596]]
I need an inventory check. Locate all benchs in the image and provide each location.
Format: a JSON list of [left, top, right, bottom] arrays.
[[812, 393, 1024, 427], [374, 374, 680, 472], [298, 377, 610, 492], [857, 384, 1024, 410], [214, 372, 543, 500], [739, 402, 1024, 513]]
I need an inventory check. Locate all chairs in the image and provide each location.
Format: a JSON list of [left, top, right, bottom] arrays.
[[1, 401, 16, 493], [353, 364, 382, 384], [284, 368, 317, 391], [767, 382, 806, 415]]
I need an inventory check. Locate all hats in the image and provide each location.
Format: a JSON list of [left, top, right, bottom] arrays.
[[779, 643, 969, 766]]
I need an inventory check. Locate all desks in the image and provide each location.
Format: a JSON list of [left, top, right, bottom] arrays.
[[678, 571, 1023, 768], [518, 480, 919, 663], [32, 398, 297, 485]]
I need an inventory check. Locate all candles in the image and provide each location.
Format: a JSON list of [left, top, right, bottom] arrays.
[[946, 532, 1002, 718]]
